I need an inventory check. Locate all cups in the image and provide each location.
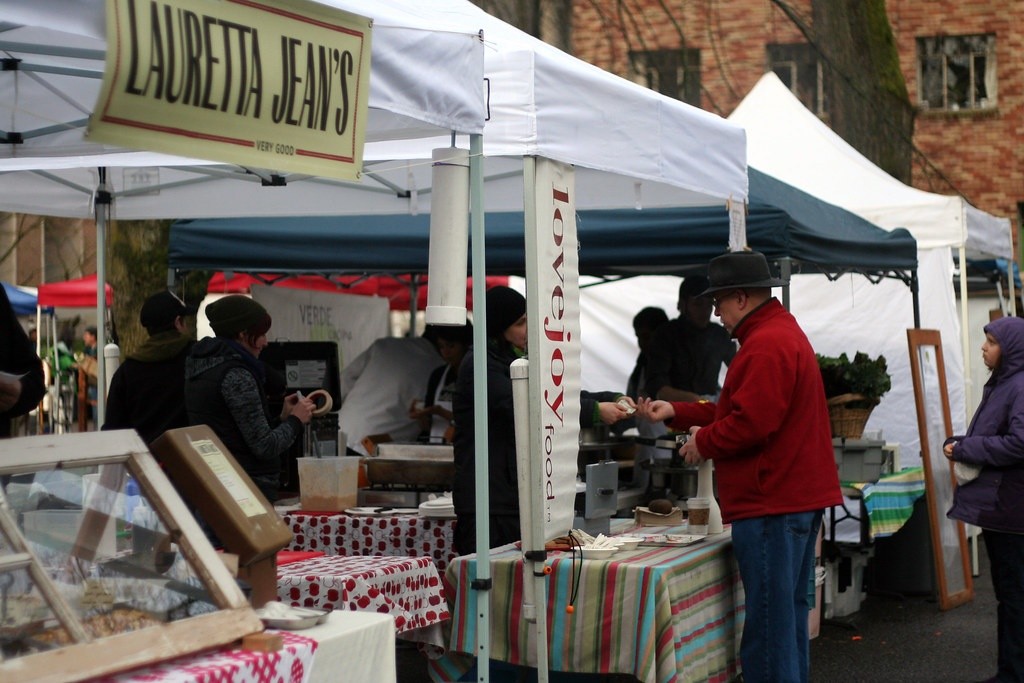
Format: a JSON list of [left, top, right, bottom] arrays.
[[686, 498, 711, 535], [676, 434, 691, 452]]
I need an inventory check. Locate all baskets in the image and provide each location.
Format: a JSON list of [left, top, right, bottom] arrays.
[[827, 392, 881, 438]]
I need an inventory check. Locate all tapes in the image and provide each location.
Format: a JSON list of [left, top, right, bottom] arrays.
[[305, 390, 333, 417]]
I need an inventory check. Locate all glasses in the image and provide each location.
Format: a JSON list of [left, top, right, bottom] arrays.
[[710, 289, 749, 309]]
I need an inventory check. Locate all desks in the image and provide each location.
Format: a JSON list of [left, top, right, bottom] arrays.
[[420, 511, 748, 683], [77, 484, 456, 683]]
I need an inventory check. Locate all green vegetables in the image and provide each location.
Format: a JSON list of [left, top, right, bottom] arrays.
[[816, 350, 891, 408]]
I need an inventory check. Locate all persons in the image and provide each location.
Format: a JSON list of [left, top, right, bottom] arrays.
[[337, 316, 475, 455], [456, 284, 638, 547], [27, 325, 98, 431], [635, 250, 843, 682], [100, 290, 319, 547], [941, 316, 1024, 682], [0, 282, 47, 489], [620, 274, 739, 499]]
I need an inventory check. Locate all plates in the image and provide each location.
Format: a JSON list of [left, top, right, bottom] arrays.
[[419, 501, 455, 516], [344, 506, 397, 515], [395, 508, 419, 513], [610, 534, 708, 547]]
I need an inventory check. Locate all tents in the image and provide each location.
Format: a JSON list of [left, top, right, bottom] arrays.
[[507, 70, 1018, 578], [160, 163, 927, 683], [0, 0, 490, 683], [0, 0, 747, 254], [35, 253, 509, 433], [0, 280, 60, 436]]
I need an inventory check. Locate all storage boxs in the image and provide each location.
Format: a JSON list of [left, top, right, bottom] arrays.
[[829, 437, 887, 483], [148, 424, 294, 564], [297, 454, 363, 514]]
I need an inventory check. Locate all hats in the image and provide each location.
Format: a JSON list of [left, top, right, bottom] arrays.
[[206, 296, 266, 338], [486, 285, 526, 337], [694, 251, 789, 300], [141, 291, 195, 327]]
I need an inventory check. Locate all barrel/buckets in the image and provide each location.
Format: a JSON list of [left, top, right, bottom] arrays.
[[297, 456, 360, 512]]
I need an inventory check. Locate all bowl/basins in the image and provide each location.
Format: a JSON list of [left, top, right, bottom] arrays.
[[570, 537, 645, 560]]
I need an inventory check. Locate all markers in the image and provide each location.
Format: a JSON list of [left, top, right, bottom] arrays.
[[296, 391, 312, 426]]
